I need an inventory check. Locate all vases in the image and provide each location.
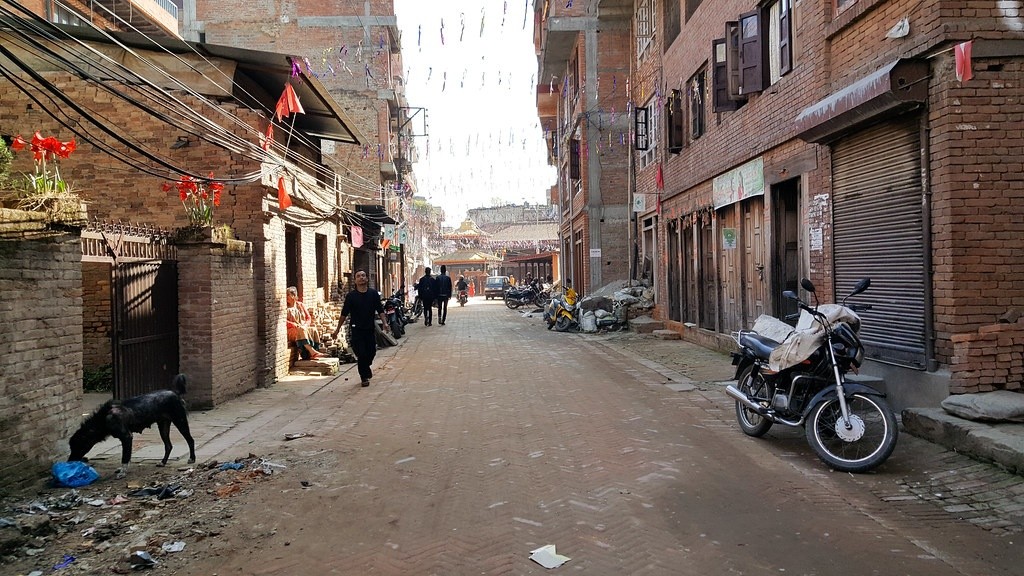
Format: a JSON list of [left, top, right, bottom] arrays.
[[2, 194, 92, 219], [168, 224, 233, 244]]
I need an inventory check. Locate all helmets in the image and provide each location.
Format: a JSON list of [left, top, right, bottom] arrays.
[[459, 275, 464, 280]]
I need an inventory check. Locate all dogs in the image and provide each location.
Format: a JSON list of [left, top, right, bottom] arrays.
[[68, 374, 196, 480]]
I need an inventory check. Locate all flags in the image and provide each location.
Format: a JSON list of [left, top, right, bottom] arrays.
[[276, 83, 305, 122], [954, 42, 972, 82], [278, 177, 292, 211]]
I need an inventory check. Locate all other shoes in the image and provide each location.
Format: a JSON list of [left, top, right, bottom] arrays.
[[425, 318, 428, 325], [429, 324, 432, 326], [362, 381, 370, 386], [438, 320, 445, 325], [368, 367, 372, 379]]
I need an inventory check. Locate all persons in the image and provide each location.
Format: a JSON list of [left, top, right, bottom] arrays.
[[510, 275, 514, 284], [455, 275, 469, 302], [418, 267, 440, 326], [469, 279, 474, 297], [435, 265, 452, 325], [286, 286, 324, 358], [332, 268, 389, 387], [525, 272, 532, 284]]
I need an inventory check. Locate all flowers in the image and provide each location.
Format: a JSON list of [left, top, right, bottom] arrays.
[[162, 170, 226, 226], [10, 129, 78, 196]]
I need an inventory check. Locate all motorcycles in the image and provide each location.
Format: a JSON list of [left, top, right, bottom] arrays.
[[725, 278, 900, 475], [381, 288, 409, 339], [456, 282, 469, 307]]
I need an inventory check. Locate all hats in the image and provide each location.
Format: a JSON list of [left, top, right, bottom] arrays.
[[527, 272, 530, 274]]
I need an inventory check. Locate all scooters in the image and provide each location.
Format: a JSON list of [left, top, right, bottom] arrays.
[[543, 278, 582, 332], [408, 294, 424, 317], [503, 277, 549, 310]]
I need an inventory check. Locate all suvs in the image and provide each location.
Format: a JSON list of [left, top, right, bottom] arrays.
[[483, 276, 510, 300]]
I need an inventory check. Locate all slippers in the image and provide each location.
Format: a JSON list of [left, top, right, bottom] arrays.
[[310, 353, 322, 359]]
